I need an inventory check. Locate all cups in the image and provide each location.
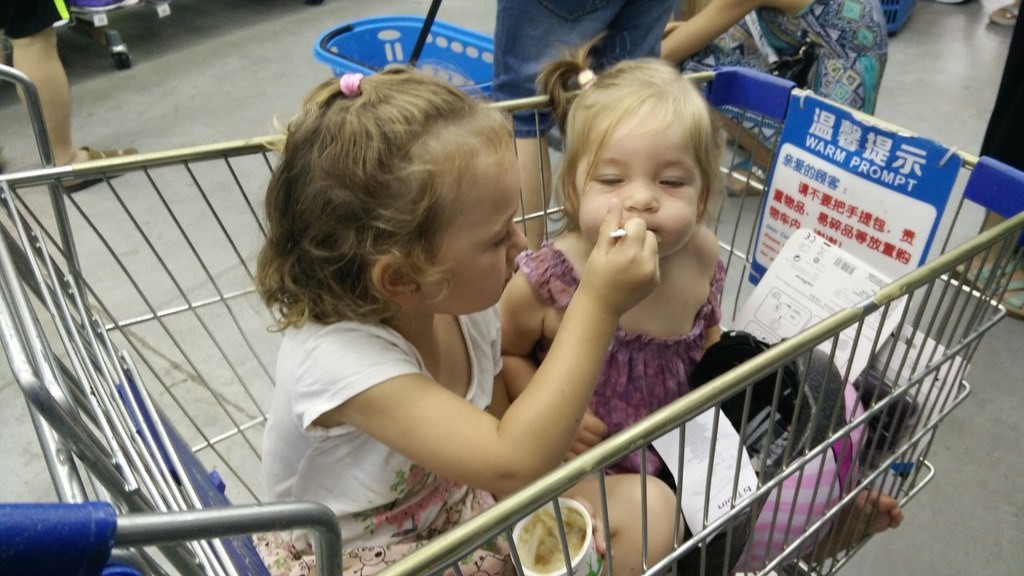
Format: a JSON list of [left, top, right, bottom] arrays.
[[508, 494, 604, 576]]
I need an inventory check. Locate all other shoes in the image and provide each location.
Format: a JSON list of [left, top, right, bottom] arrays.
[[989, 3, 1019, 25]]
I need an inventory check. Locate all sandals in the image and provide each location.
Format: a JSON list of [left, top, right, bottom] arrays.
[[56, 146, 138, 193]]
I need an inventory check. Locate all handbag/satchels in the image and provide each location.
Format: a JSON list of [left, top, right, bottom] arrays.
[[765, 42, 815, 87]]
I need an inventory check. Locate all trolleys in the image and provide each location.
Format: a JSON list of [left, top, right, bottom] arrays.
[[49, 0, 329, 70], [0, 62, 1023, 576]]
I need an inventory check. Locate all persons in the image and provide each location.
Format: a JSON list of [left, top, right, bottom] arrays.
[[662, 0, 890, 197], [261, 63, 683, 576], [0, 0, 139, 193], [500, 57, 903, 576], [936, 0, 1024, 27]]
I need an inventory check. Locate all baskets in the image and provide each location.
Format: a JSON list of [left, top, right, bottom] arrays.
[[878, 0, 914, 37], [313, 15, 496, 104]]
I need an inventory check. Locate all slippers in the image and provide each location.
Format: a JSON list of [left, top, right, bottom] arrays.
[[953, 261, 1024, 320]]
